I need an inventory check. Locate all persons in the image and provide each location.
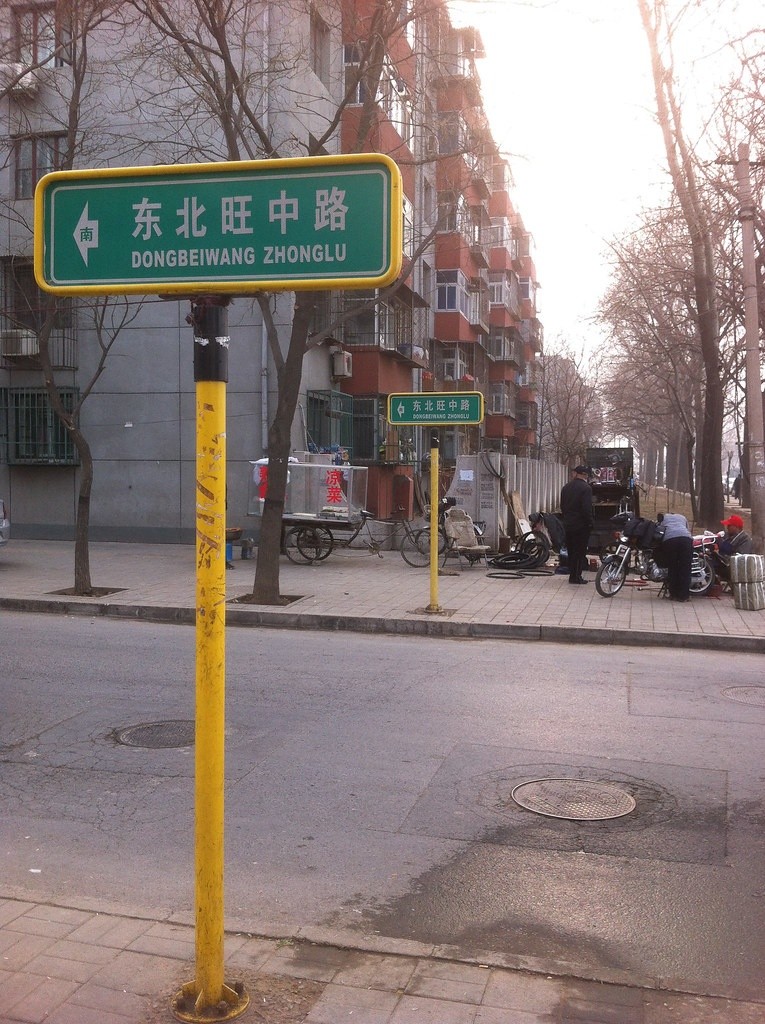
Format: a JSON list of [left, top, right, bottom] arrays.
[[561, 465, 594, 584], [652, 513, 693, 601], [700, 516, 752, 596], [733, 474, 743, 499]]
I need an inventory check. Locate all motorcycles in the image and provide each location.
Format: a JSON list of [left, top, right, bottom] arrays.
[[594, 513, 719, 601]]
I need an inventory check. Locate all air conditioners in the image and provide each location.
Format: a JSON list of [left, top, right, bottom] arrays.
[[0, 330, 39, 357], [427, 136, 439, 153], [333, 350, 353, 378], [0, 63, 39, 94]]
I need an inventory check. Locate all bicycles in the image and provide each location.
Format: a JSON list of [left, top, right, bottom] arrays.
[[415, 500, 484, 564]]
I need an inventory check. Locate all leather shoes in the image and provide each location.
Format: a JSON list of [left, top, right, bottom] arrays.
[[569, 574, 589, 584]]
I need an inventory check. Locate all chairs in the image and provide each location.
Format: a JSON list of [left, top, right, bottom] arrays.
[[441, 511, 491, 571]]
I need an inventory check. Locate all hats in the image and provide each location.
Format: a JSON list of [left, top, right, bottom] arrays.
[[720, 515, 744, 528], [577, 466, 592, 478], [571, 465, 587, 472]]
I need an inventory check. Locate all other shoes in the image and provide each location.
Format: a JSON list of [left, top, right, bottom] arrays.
[[671, 592, 689, 601]]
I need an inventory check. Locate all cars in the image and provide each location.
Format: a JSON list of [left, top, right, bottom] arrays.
[[722, 478, 736, 497]]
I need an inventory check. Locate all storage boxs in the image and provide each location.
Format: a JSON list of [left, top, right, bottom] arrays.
[[730, 554, 765, 583], [732, 581, 765, 611]]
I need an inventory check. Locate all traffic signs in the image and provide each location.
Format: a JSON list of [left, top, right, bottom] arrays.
[[34, 153, 406, 295], [384, 390, 484, 427]]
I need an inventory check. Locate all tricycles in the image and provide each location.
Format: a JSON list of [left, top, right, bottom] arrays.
[[281, 511, 431, 567]]
